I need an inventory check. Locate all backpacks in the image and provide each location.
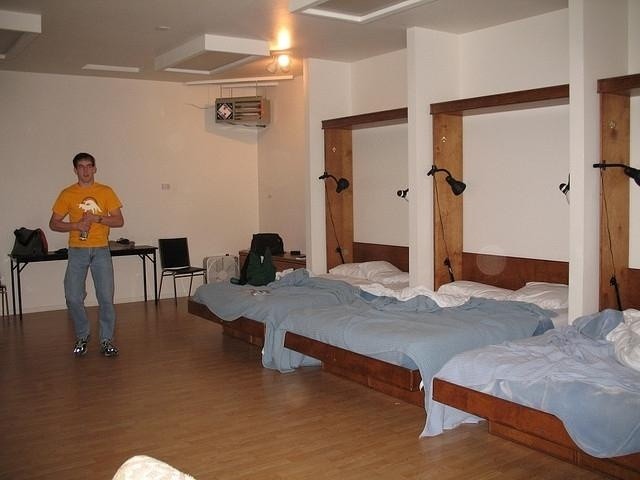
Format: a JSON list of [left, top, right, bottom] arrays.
[[230, 246, 276, 286]]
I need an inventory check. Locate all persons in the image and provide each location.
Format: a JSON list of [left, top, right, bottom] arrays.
[[49, 153, 125, 356]]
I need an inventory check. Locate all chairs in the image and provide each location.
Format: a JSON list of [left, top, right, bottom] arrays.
[[157, 237, 209, 308]]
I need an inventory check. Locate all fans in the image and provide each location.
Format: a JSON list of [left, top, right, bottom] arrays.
[[202, 253, 238, 286]]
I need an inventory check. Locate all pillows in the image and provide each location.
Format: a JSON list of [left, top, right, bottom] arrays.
[[509, 280, 569, 311], [358, 259, 410, 287], [328, 261, 366, 281], [437, 280, 517, 308]]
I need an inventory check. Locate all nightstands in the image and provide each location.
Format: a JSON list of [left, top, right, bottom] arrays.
[[238, 248, 307, 278]]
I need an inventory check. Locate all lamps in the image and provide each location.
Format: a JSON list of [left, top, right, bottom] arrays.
[[318, 171, 350, 264], [265, 54, 277, 74], [427, 165, 466, 283], [593, 163, 640, 311]]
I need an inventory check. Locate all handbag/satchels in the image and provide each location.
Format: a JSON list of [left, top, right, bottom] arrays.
[[12, 228, 48, 260]]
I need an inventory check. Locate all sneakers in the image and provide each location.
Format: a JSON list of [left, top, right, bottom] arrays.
[[101, 339, 119, 357], [73, 335, 91, 357]]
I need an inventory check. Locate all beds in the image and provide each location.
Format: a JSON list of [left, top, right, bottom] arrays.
[[181, 244, 412, 348], [288, 251, 571, 410], [433, 268, 640, 480]]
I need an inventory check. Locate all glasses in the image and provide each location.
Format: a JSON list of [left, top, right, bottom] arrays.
[[79, 165, 93, 169]]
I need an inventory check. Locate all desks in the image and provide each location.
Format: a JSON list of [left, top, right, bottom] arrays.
[[7, 246, 158, 321]]
